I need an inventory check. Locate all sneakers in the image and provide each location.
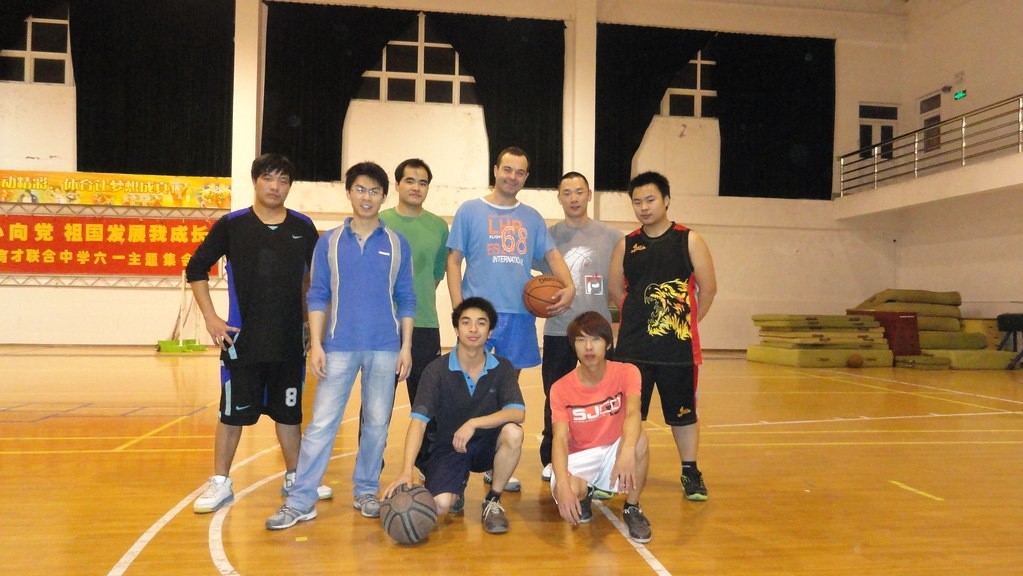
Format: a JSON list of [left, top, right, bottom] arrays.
[[280, 471, 333, 500], [622, 506, 653, 544], [450, 491, 465, 511], [578, 485, 595, 522], [264, 503, 319, 530], [193, 475, 234, 514], [681, 469, 708, 500], [354, 494, 381, 517], [482, 499, 510, 534]]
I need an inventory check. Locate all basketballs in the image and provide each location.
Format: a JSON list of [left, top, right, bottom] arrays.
[[523, 275, 564, 318], [847, 354, 863, 369], [380, 483, 437, 544]]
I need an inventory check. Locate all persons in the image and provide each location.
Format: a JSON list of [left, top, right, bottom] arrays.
[[444, 146, 576, 494], [358, 158, 450, 484], [608, 171, 717, 501], [380, 297, 526, 535], [265, 163, 417, 531], [530, 171, 627, 481], [550, 311, 654, 543], [183, 153, 332, 514]]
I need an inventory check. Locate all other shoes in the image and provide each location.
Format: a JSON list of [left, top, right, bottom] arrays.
[[542, 463, 553, 481], [483, 469, 521, 491], [592, 488, 614, 499]]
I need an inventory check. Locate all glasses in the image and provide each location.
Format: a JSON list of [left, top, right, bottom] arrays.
[[353, 186, 381, 196]]
